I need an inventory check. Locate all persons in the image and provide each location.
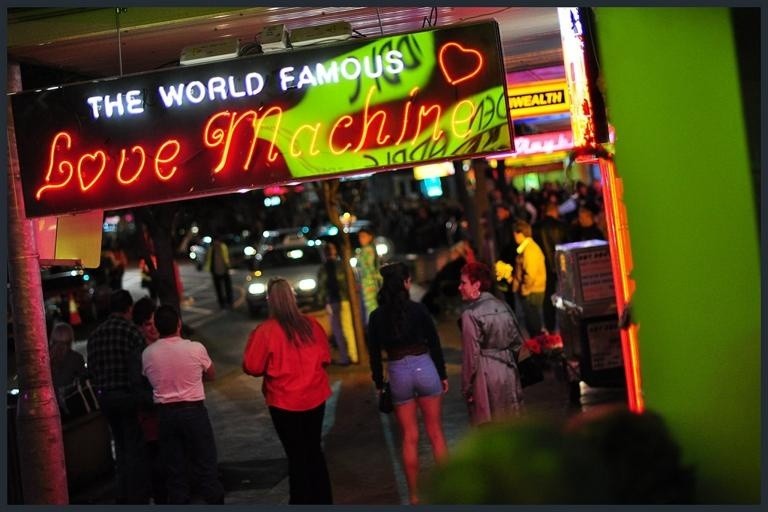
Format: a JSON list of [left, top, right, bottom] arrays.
[[510, 180, 603, 218], [204, 236, 235, 309], [46, 305, 62, 341], [368, 262, 449, 505], [88, 289, 148, 504], [457, 261, 524, 428], [568, 208, 605, 244], [356, 229, 382, 329], [510, 217, 546, 339], [488, 189, 517, 264], [132, 295, 161, 345], [141, 302, 225, 507], [48, 322, 91, 393], [103, 236, 124, 289], [242, 276, 332, 505], [533, 204, 572, 333], [317, 242, 358, 366], [90, 268, 114, 327]]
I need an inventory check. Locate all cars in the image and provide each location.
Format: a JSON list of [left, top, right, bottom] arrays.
[[245, 243, 329, 313]]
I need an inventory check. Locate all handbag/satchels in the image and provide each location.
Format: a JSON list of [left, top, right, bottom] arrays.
[[479, 299, 544, 389], [378, 383, 393, 413]]
